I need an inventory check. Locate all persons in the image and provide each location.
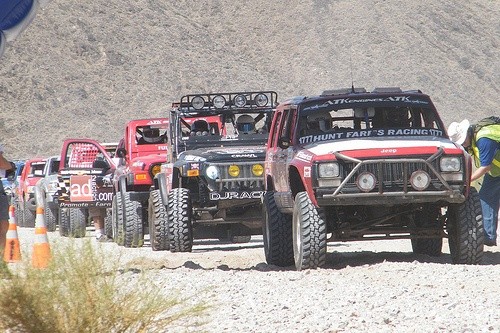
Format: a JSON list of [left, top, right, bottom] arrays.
[[448, 115, 499, 246], [137, 110, 336, 144], [0, 146, 17, 281]]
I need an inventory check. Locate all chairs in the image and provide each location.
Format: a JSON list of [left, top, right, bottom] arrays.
[[91, 105, 411, 167]]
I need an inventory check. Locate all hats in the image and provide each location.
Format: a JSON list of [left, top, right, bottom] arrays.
[[447, 119, 472, 144]]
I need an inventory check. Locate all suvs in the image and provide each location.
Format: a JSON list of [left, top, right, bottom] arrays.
[[110, 116, 234, 248], [261, 84, 485, 270], [147, 92, 283, 252], [2, 136, 125, 239]]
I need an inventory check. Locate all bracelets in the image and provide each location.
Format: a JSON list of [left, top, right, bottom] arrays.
[[9, 161, 15, 172]]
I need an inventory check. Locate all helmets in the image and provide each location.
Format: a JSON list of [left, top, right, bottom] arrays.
[[143, 125, 161, 142], [307, 110, 333, 133], [236, 115, 256, 136]]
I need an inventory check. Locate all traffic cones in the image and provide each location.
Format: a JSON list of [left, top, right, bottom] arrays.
[[1, 205, 27, 263], [26, 206, 56, 270]]
